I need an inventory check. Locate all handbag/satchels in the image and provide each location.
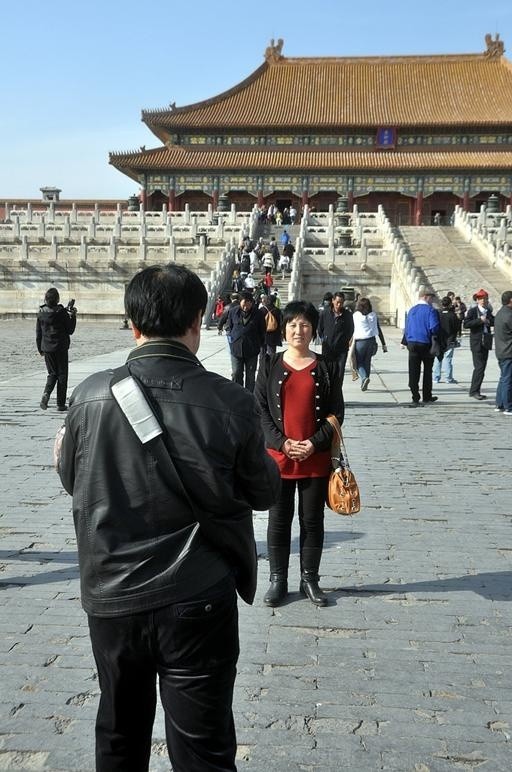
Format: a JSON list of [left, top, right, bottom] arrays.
[[309, 329, 324, 356], [429, 335, 442, 358], [323, 415, 361, 515], [481, 332, 494, 351]]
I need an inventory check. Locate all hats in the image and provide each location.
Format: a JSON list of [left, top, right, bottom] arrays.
[[475, 289, 489, 297], [418, 288, 435, 297]]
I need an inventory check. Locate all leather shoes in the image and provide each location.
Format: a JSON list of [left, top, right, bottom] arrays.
[[469, 394, 486, 400]]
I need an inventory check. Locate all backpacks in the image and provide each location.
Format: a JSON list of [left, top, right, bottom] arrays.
[[263, 311, 278, 332]]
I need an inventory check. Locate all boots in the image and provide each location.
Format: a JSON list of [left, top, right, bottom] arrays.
[[263, 571, 288, 608], [298, 569, 328, 608]]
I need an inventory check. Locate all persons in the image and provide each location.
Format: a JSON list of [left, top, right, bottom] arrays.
[[252, 299, 344, 607], [36, 287, 78, 411], [404, 290, 512, 416], [257, 203, 297, 226], [317, 291, 388, 390], [215, 231, 296, 390], [57, 264, 283, 772]]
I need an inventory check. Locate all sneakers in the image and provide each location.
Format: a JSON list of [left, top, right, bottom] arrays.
[[432, 375, 457, 385], [411, 397, 438, 404], [200, 509, 260, 607], [39, 396, 48, 410], [494, 406, 512, 416], [57, 404, 69, 411], [351, 371, 359, 381], [360, 377, 370, 393]]
